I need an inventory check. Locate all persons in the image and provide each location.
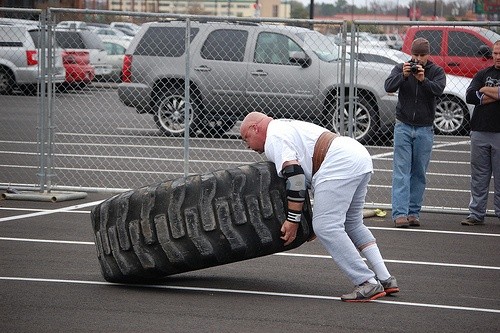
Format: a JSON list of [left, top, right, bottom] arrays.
[[461, 41, 500, 227], [384, 38, 446, 227], [241, 111, 399, 302]]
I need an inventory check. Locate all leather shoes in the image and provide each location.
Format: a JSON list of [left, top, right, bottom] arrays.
[[395, 216, 409, 227], [408, 215, 419, 226]]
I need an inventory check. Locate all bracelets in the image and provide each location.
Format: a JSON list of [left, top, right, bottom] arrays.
[[288, 209, 301, 223]]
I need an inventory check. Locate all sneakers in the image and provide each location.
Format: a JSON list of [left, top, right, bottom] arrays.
[[377, 276, 399, 293], [340, 282, 386, 304]]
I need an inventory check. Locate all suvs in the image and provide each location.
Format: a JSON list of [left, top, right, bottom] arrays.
[[117, 19, 500, 145]]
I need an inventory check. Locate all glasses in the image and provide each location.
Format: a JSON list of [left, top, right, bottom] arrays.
[[242, 125, 252, 142]]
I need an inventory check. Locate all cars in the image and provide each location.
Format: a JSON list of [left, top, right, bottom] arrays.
[[0, 20, 141, 95]]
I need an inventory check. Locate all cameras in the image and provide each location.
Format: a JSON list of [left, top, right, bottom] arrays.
[[410, 62, 421, 74]]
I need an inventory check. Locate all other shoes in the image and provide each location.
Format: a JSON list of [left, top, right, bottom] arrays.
[[461, 217, 483, 226]]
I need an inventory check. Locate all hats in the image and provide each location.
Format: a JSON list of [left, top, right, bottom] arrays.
[[411, 38, 430, 55]]
